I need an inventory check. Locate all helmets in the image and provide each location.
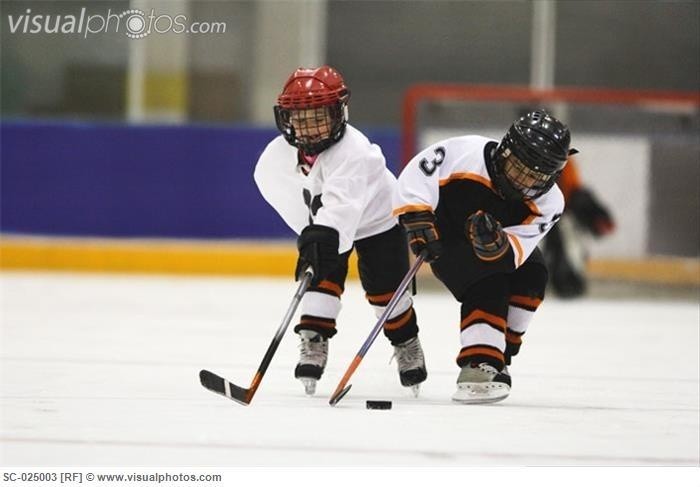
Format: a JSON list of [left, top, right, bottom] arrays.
[[495, 109, 570, 174], [274, 64, 351, 109]]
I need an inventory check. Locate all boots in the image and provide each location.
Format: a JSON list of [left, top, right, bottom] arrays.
[[393, 335, 427, 387], [295, 329, 328, 380], [456, 359, 511, 389]]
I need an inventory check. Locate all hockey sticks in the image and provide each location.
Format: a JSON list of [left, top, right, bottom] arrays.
[[199, 267, 315, 406], [329, 248, 430, 405]]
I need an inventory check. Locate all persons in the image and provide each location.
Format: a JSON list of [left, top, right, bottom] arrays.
[[254, 67, 614, 386]]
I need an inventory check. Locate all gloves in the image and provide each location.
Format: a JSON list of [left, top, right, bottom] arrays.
[[399, 211, 442, 263], [295, 224, 339, 287], [464, 209, 510, 263]]
